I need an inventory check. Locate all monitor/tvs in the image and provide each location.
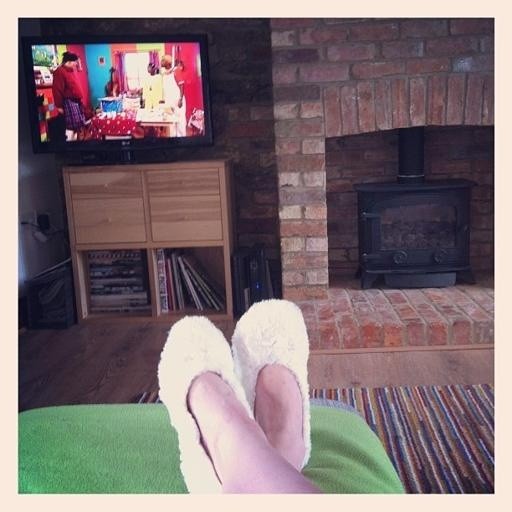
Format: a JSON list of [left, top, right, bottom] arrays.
[[21, 34, 215, 162]]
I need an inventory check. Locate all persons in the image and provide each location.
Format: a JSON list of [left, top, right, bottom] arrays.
[[142, 64, 162, 137], [160, 56, 187, 137], [157, 299, 325, 495], [52, 54, 85, 139], [106, 68, 120, 98]]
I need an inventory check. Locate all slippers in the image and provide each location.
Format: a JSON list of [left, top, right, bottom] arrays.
[[157, 316, 253, 495], [232, 299, 310, 472]]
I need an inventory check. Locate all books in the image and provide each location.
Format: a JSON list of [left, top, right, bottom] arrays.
[[232, 252, 280, 320], [87, 250, 152, 313], [155, 249, 226, 312]]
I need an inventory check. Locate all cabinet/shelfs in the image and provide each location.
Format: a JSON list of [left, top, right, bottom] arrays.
[[61, 158, 234, 326]]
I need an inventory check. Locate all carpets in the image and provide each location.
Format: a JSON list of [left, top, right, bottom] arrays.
[[138, 382, 494, 494]]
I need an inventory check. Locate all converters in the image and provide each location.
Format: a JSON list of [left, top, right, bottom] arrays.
[[37, 213, 51, 230]]
